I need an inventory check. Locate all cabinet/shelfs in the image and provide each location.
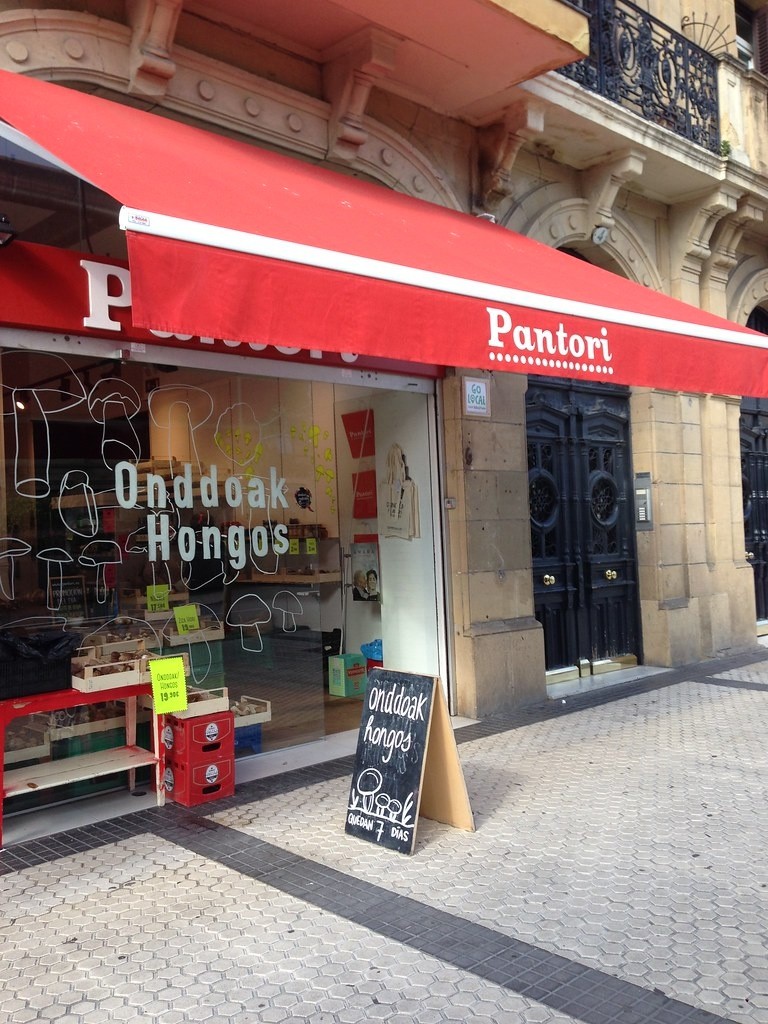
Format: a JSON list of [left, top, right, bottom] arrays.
[[0, 684, 166, 851], [221, 536, 342, 583]]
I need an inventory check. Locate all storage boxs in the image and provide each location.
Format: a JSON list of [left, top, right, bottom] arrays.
[[328, 653, 368, 697], [51, 640, 261, 807]]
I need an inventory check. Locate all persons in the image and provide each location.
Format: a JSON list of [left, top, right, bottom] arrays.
[[124, 522, 182, 596], [353, 570, 369, 601], [87, 543, 113, 617], [367, 569, 380, 601], [178, 513, 226, 618]]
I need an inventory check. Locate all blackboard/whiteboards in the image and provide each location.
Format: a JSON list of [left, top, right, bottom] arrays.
[[345, 666, 474, 856]]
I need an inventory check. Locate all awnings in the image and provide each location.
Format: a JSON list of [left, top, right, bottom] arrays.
[[0, 71, 768, 398]]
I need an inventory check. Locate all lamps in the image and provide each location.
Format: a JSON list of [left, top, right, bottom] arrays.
[[0, 213, 18, 247]]
